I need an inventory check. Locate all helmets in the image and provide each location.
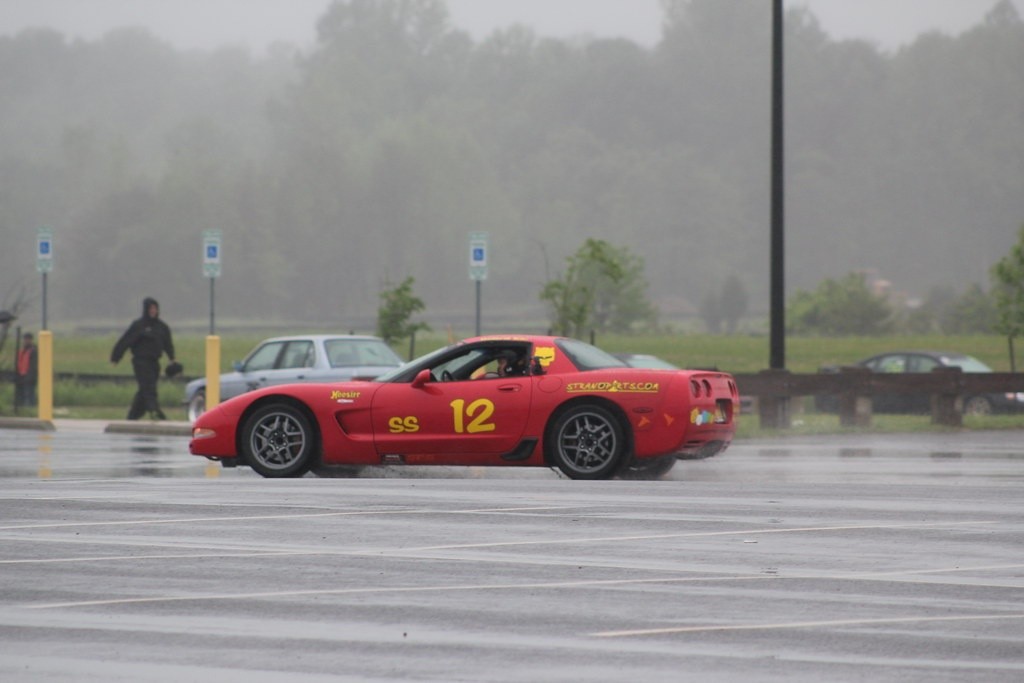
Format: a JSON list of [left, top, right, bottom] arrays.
[[497, 350, 520, 377]]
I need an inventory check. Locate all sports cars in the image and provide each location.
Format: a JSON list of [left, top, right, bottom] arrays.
[[189, 334, 736, 480]]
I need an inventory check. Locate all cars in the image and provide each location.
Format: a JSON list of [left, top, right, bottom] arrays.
[[183, 336, 408, 421], [817, 351, 1024, 414]]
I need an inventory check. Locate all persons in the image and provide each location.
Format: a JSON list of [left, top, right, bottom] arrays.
[[495, 350, 518, 377], [110, 297, 174, 421], [15, 332, 38, 405]]
